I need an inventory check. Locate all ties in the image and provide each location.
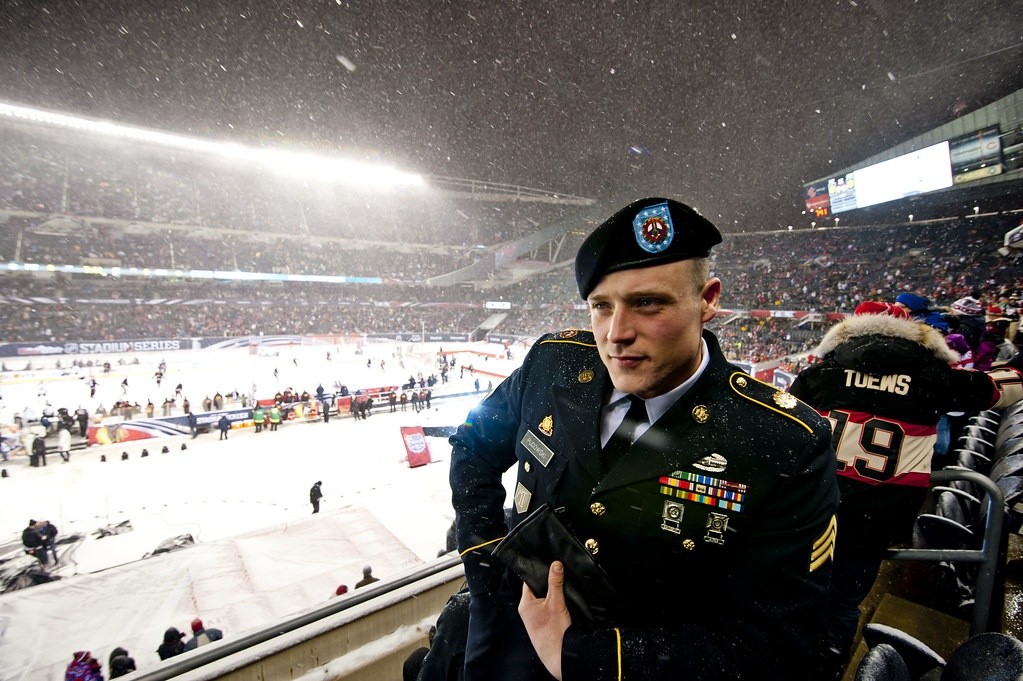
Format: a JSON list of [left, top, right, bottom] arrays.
[[601, 395, 647, 478]]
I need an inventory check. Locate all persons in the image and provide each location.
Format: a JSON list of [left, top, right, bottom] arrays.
[[402, 197, 837, 681], [327, 585, 348, 598], [705, 179, 1023, 610], [435, 508, 512, 559], [64, 619, 222, 681], [0, 129, 594, 467], [403, 587, 471, 681], [22, 519, 59, 566], [310, 480, 322, 514], [355, 566, 381, 588]]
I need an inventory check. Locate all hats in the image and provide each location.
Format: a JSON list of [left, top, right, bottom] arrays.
[[337, 584, 349, 595], [950, 293, 982, 313], [896, 292, 929, 311], [854, 301, 909, 321], [575, 197, 722, 300], [72, 650, 91, 662]]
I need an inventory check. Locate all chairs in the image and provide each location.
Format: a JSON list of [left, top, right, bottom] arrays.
[[850, 396, 1023, 680]]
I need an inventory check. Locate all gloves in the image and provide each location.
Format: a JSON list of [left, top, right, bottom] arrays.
[[491, 502, 623, 632]]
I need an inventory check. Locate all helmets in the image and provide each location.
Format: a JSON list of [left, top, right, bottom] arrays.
[[191, 618, 203, 632]]
